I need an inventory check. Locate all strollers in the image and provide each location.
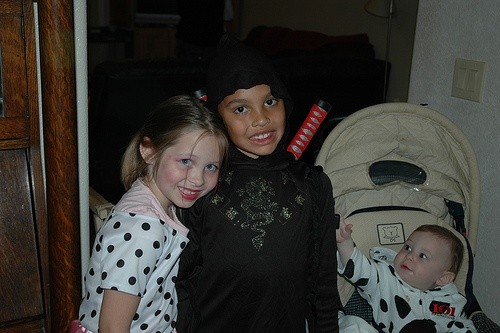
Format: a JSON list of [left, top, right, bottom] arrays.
[[312, 102, 500, 333]]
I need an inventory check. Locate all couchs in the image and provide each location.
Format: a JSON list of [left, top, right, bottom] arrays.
[[93, 25, 391, 181]]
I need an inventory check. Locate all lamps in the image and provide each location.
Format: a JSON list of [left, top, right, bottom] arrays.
[[364, 1, 397, 101]]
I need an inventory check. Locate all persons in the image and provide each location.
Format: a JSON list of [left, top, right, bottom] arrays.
[[78, 95, 228, 333], [177, 43, 338, 333], [335, 208, 478, 333]]
